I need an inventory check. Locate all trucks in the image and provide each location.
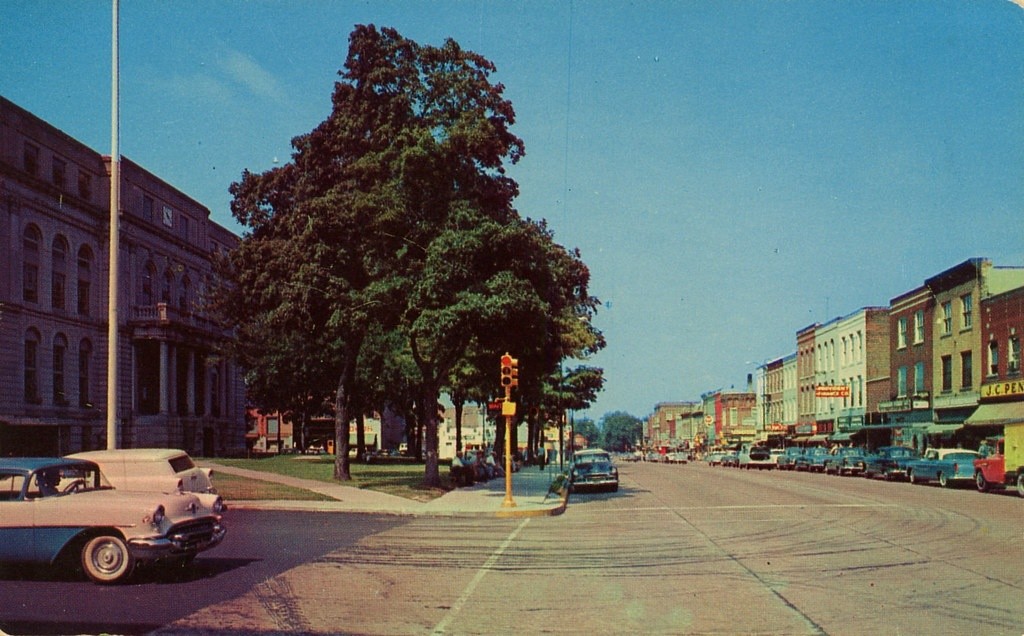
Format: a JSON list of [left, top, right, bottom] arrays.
[[973, 417, 1024, 498], [737, 444, 776, 471]]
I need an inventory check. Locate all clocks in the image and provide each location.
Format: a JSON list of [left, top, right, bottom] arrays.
[[162, 205, 173, 229]]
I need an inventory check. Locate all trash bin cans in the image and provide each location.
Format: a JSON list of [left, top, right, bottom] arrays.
[[547, 448, 558, 462]]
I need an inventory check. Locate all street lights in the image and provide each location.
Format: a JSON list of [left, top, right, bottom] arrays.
[[680, 400, 692, 462], [745, 361, 766, 431]]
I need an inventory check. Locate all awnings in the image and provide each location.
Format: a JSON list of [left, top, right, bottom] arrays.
[[831, 432, 857, 440], [793, 436, 807, 441], [966, 402, 1024, 425], [809, 435, 829, 441], [926, 423, 964, 434]]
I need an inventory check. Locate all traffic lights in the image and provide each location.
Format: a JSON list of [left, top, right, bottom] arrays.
[[487, 402, 502, 417], [501, 355, 512, 386], [512, 359, 519, 386]]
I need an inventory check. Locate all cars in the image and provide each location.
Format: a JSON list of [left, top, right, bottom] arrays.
[[568, 448, 619, 491], [0, 457, 228, 586], [864, 446, 922, 481], [794, 448, 833, 472], [905, 448, 982, 487], [620, 452, 737, 468], [823, 447, 867, 476], [776, 447, 801, 470], [770, 449, 786, 467]]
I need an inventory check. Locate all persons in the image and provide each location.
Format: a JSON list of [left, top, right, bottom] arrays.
[[36, 468, 60, 498], [485, 450, 505, 477], [979, 440, 994, 455], [517, 447, 534, 468], [537, 443, 545, 470], [452, 451, 475, 486], [481, 452, 495, 480], [470, 451, 487, 482]]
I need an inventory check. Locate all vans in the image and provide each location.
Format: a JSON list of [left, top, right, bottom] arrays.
[[0, 448, 218, 494]]
